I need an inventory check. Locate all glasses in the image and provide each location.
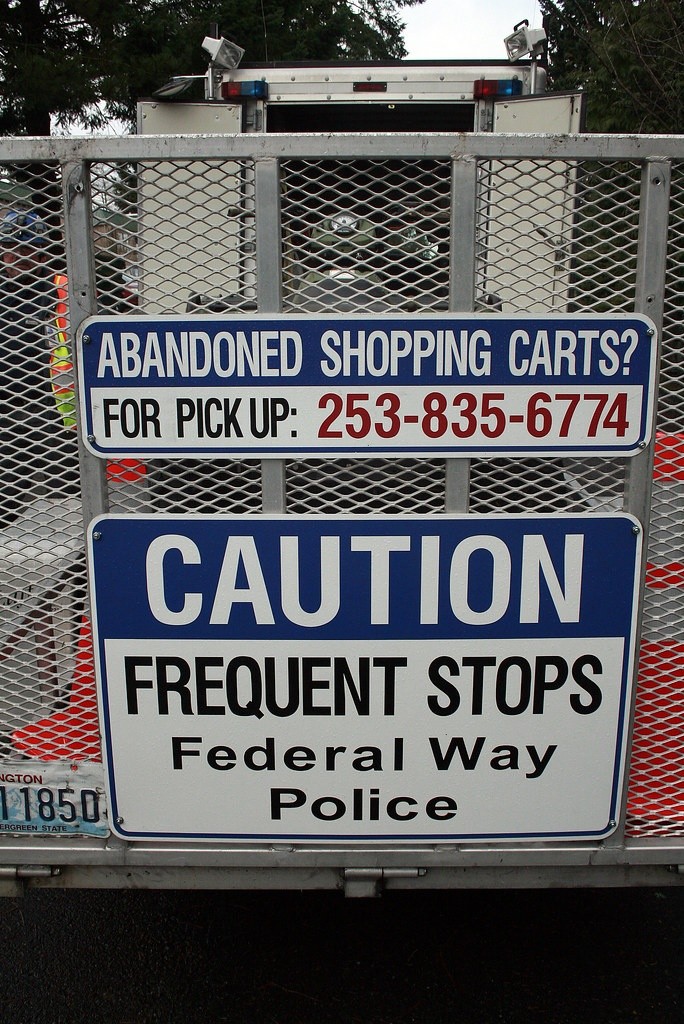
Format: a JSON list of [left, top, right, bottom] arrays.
[[2, 244, 39, 253]]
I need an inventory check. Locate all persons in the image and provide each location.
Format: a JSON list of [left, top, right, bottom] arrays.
[[0, 208, 84, 529]]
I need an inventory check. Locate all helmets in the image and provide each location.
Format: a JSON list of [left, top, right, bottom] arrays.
[[0, 208, 50, 244]]
